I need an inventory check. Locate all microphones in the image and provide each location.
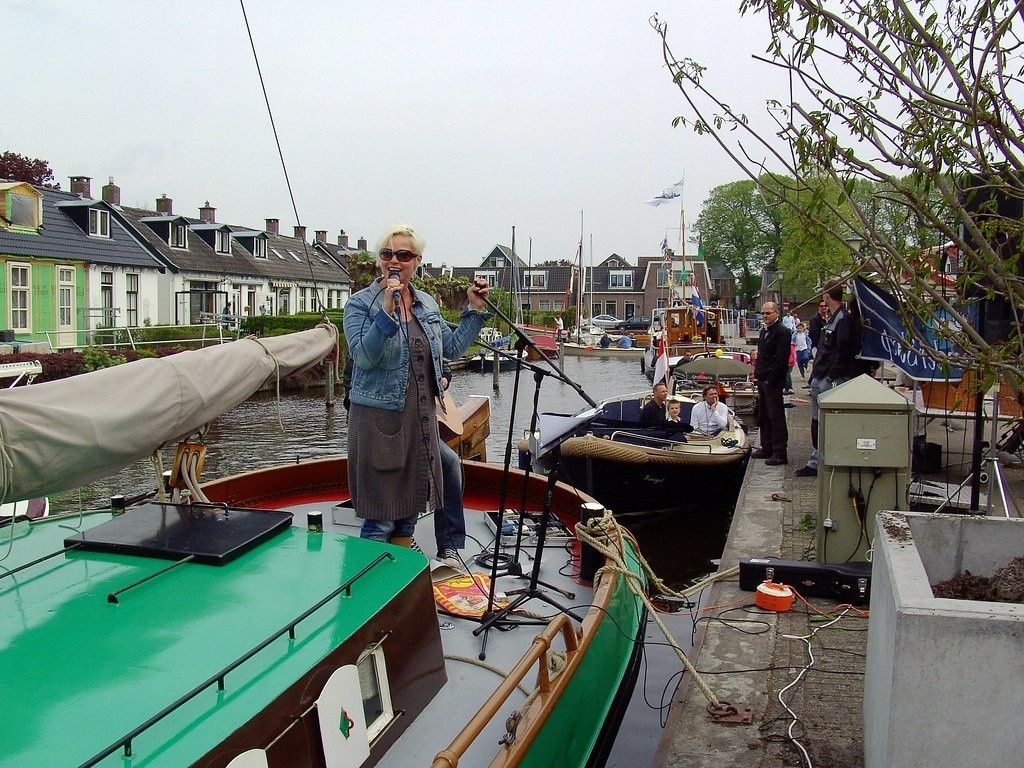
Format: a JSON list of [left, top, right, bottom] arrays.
[[388, 271, 400, 305]]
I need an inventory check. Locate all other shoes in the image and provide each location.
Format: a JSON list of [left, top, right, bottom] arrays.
[[765, 457, 788, 465], [795, 466, 818, 475], [783, 388, 794, 395], [752, 450, 771, 458], [801, 383, 812, 388]]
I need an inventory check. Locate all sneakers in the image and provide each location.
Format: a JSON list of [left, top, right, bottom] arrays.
[[436, 548, 460, 567]]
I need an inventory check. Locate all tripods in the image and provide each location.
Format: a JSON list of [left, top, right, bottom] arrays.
[[933, 392, 1024, 518], [437, 338, 585, 661]]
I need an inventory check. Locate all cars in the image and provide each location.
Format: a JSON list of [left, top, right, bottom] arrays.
[[615, 316, 652, 333], [584, 314, 626, 330]]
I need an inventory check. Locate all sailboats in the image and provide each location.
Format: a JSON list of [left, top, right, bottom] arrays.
[[470, 226, 529, 374], [0, 1, 650, 768]]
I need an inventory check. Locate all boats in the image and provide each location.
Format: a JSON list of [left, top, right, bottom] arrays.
[[524, 335, 560, 361], [639, 199, 759, 417], [549, 389, 750, 520], [556, 210, 647, 360]]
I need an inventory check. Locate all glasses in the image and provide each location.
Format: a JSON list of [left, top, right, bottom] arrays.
[[378, 248, 419, 262], [761, 310, 777, 315], [819, 305, 829, 307]]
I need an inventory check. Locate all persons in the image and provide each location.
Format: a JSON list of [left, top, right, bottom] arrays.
[[675, 350, 693, 381], [690, 386, 727, 436], [639, 381, 668, 426], [343, 350, 468, 569], [746, 347, 757, 379], [783, 311, 813, 394], [617, 332, 632, 348], [341, 225, 495, 550], [664, 400, 695, 432], [749, 301, 791, 465], [554, 315, 564, 342], [223, 302, 231, 331], [793, 280, 880, 478], [599, 333, 610, 348]]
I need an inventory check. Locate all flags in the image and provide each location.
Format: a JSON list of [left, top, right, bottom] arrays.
[[651, 335, 668, 389], [690, 280, 703, 325]]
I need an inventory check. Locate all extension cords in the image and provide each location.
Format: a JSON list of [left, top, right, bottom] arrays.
[[822, 519, 833, 527]]
